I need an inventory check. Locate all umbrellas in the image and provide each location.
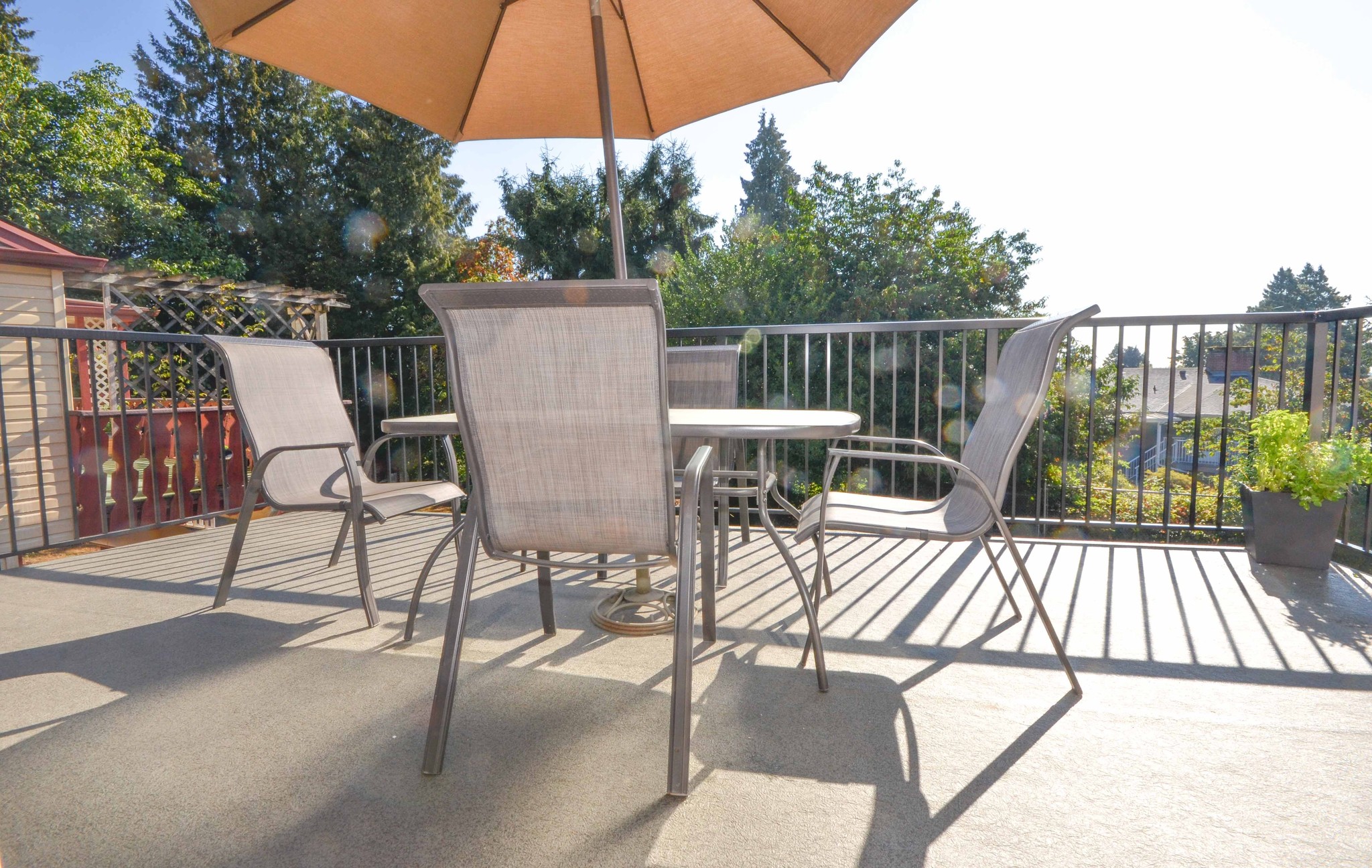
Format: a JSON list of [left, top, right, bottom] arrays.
[[187, 0, 919, 595]]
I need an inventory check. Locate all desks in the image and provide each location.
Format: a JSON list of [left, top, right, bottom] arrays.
[[377, 406, 864, 696]]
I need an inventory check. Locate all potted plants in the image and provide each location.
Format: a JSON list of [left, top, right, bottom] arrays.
[[1232, 403, 1372, 574]]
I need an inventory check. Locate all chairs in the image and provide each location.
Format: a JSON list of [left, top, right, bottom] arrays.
[[789, 304, 1105, 699], [417, 277, 721, 808], [598, 345, 752, 587], [200, 333, 467, 631]]
